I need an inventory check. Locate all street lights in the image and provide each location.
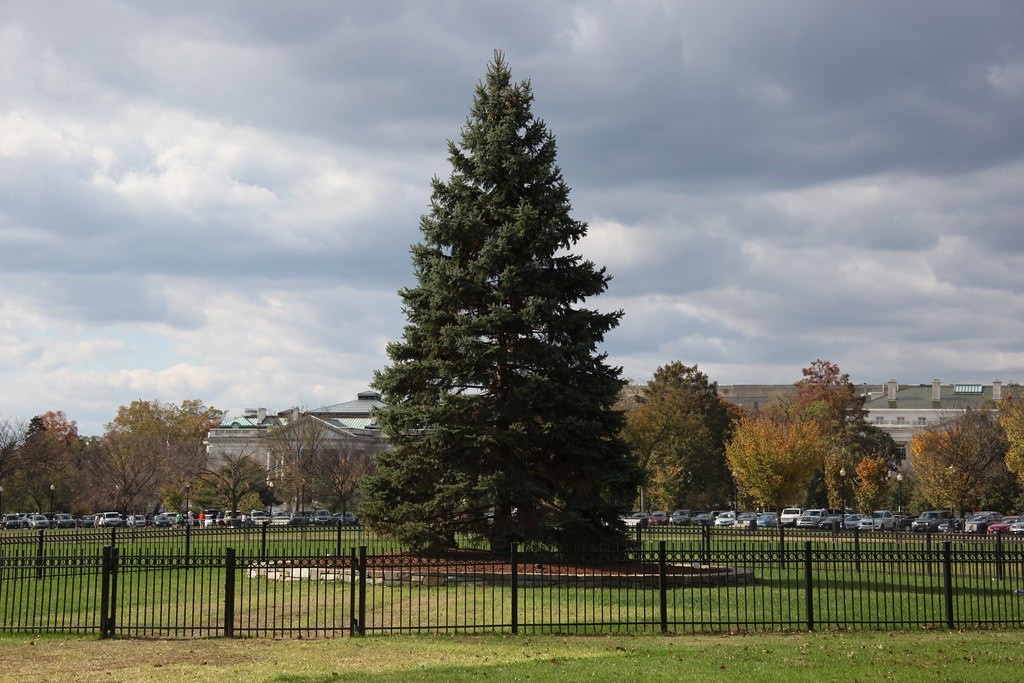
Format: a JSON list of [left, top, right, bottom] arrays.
[[185, 482, 191, 533], [49, 484, 55, 529], [897, 473, 903, 519], [839, 468, 847, 530], [269, 481, 274, 516]]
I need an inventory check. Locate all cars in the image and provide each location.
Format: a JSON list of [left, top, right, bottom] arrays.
[[0, 506, 360, 530], [630, 508, 1024, 537]]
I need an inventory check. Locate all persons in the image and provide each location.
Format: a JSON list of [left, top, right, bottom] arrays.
[[131, 513, 253, 528], [2, 515, 107, 529]]
